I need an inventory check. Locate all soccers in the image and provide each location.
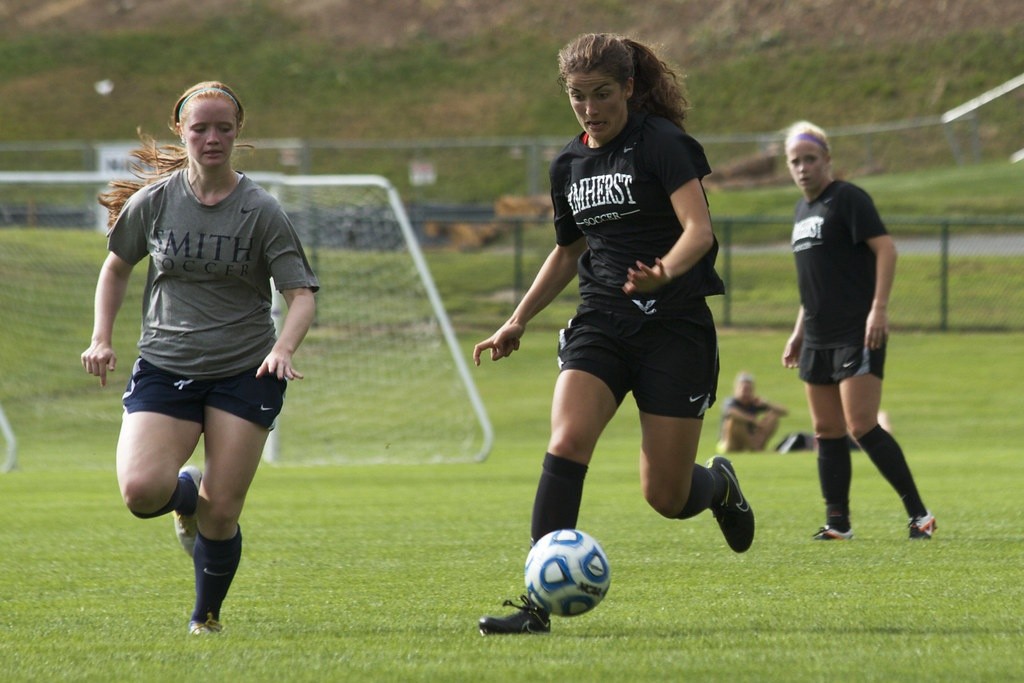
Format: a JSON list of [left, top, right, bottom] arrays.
[[524, 528, 612, 617]]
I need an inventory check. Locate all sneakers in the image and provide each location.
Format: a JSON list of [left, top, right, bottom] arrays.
[[704, 455, 755, 554], [908, 513, 938, 542], [172, 465, 203, 558], [479, 595, 551, 636], [189, 612, 220, 639], [812, 525, 855, 540]]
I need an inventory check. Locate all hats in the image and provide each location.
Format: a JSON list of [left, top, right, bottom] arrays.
[[736, 372, 755, 383]]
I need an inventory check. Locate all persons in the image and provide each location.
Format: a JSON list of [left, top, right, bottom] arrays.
[[472, 31, 757, 636], [780, 121, 939, 542], [79, 79, 321, 636], [717, 373, 790, 453]]
